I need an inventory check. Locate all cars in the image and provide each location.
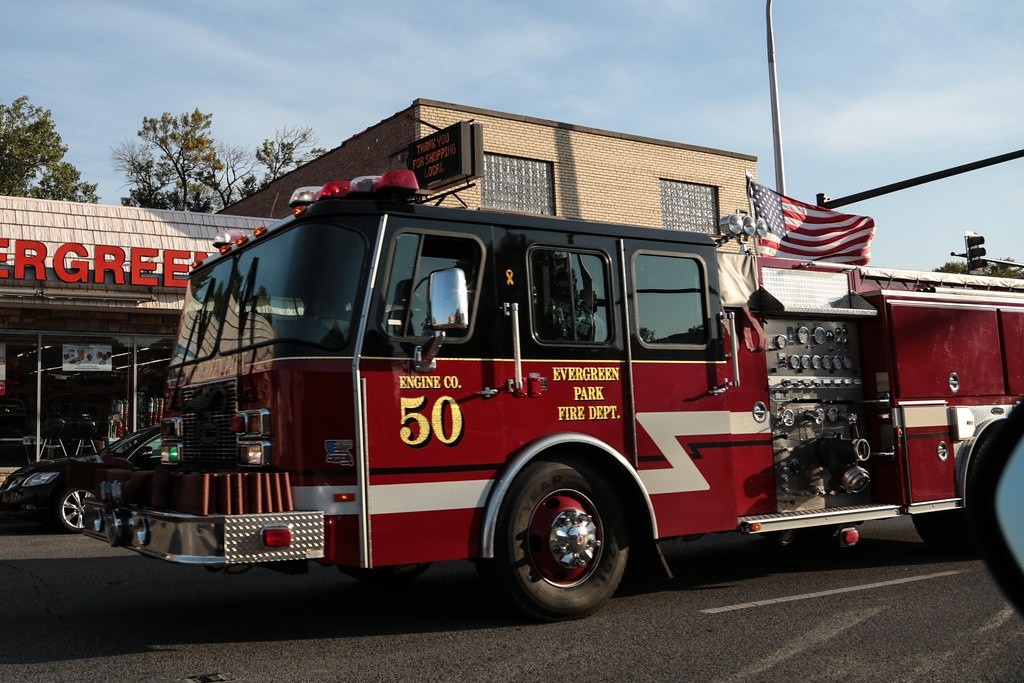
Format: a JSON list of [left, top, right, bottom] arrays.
[[0, 424, 165, 535]]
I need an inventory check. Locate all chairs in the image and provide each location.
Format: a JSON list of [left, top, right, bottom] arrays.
[[41, 418, 69, 457], [74, 421, 99, 455]]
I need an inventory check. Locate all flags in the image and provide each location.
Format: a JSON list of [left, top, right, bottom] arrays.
[[746, 175, 875, 267]]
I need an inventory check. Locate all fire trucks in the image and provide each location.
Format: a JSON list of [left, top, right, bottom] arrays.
[[81, 117, 1024, 621]]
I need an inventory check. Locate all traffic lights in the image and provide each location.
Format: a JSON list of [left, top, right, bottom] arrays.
[[963, 231, 988, 274]]
